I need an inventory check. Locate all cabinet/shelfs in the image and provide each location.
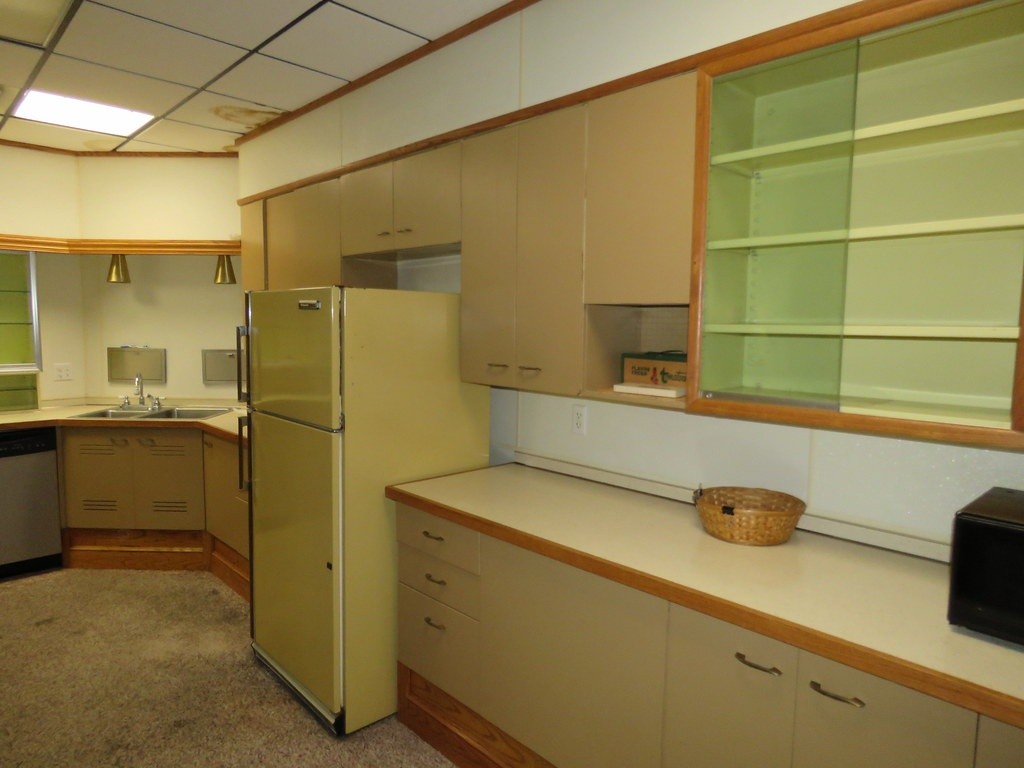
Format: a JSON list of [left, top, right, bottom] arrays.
[[340, 130, 460, 262], [396, 502, 668, 768], [459, 115, 641, 396], [660, 601, 1024, 768], [581, 57, 697, 411], [265, 167, 397, 288], [237, 191, 265, 292], [62, 425, 205, 571], [686, 1, 1024, 450], [203, 430, 251, 603]]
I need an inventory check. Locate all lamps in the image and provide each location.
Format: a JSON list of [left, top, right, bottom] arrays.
[[214, 255, 236, 284], [107, 254, 130, 284]]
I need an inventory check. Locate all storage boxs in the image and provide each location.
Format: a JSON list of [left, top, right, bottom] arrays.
[[620, 351, 688, 388]]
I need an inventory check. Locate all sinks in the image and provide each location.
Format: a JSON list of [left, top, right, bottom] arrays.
[[139, 408, 230, 419]]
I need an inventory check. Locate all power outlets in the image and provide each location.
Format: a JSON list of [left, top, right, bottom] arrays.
[[53, 362, 73, 381], [572, 404, 588, 435]]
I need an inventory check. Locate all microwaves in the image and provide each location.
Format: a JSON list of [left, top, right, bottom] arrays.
[[948, 486, 1024, 646]]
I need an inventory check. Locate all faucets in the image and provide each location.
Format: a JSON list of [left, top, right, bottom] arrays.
[[134, 371, 145, 405], [68, 409, 148, 419]]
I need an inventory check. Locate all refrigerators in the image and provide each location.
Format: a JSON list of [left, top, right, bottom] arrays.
[[235, 288, 493, 736]]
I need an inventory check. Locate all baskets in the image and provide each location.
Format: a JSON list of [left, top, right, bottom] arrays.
[[692, 484, 810, 546]]
